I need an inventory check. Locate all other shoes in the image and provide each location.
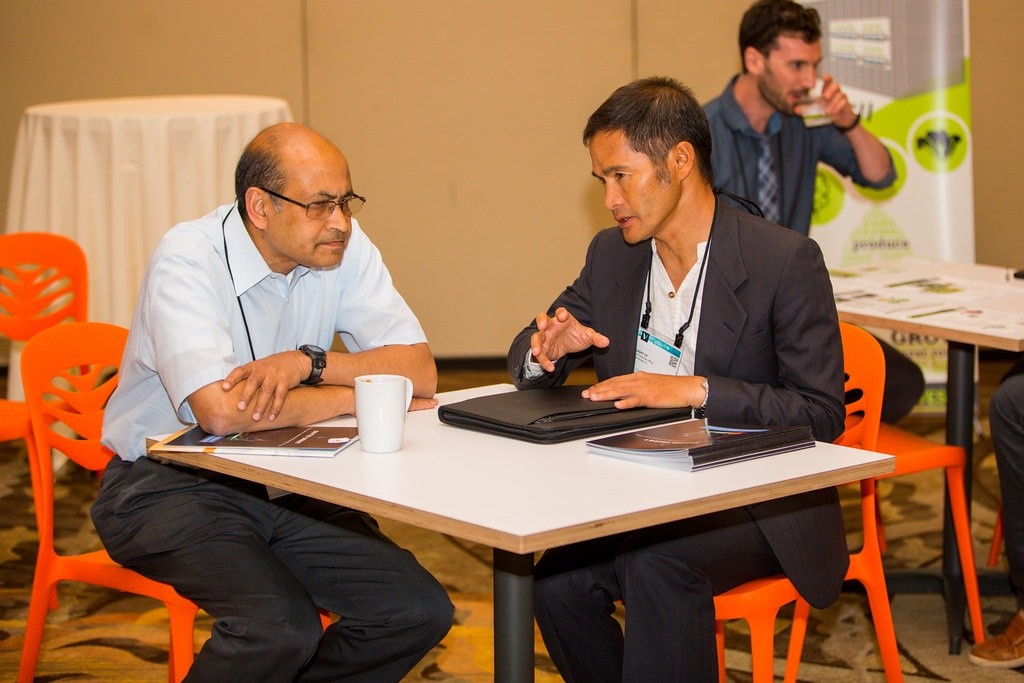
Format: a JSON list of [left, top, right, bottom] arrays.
[[969, 611, 1024, 667]]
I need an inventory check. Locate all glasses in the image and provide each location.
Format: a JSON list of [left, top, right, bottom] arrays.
[[260, 187, 366, 220], [757, 8, 822, 47]]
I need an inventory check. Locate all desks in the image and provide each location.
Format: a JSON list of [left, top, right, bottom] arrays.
[[4, 92, 294, 473], [836, 259, 1024, 656], [144, 381, 897, 683]]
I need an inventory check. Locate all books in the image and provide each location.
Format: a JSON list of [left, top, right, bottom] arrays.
[[587, 418, 815, 473], [150, 423, 359, 458]]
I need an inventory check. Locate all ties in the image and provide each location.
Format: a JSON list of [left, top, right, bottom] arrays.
[[742, 126, 781, 225]]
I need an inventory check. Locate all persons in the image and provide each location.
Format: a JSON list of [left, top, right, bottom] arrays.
[[698, 0, 925, 427], [507, 78, 851, 683], [968, 361, 1024, 667], [90, 122, 457, 683]]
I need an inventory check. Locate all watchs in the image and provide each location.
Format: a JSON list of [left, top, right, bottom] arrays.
[[833, 108, 861, 133], [298, 343, 327, 386]]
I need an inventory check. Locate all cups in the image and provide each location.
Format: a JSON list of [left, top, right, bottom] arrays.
[[794, 89, 832, 127], [355, 375, 413, 454]]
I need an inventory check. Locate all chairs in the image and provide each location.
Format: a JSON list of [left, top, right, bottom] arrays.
[[714, 323, 903, 683], [843, 412, 986, 645], [0, 231, 105, 481], [17, 321, 333, 683]]
